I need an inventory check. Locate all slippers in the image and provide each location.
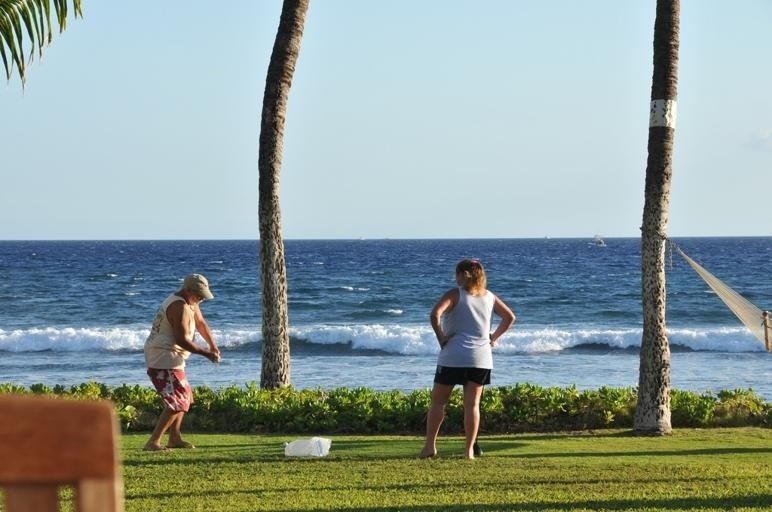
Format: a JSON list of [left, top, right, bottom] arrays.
[[143, 445, 169, 451], [166, 441, 195, 449]]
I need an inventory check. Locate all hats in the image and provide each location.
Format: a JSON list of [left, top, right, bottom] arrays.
[[184, 273, 214, 300]]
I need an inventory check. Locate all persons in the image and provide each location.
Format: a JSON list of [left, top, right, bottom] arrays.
[[141, 274, 221, 451], [420, 260, 515, 460]]
